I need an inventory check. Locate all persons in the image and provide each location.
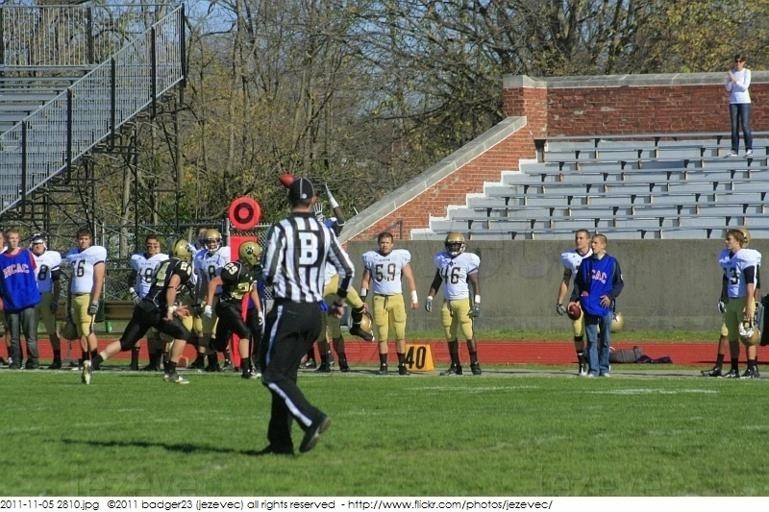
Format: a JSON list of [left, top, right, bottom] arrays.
[[556, 229, 596, 376], [700, 228, 749, 377], [722, 230, 760, 379], [566, 234, 623, 376], [426, 231, 482, 375], [724, 54, 753, 159], [257, 176, 354, 455]]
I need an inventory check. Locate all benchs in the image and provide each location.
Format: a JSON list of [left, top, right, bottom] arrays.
[[0, 63, 183, 211], [410, 131, 769, 239]]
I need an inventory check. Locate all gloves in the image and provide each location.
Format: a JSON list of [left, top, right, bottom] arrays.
[[130, 291, 142, 306], [203, 304, 212, 320], [556, 303, 566, 316], [88, 301, 99, 315], [257, 311, 264, 326], [467, 302, 480, 318], [717, 298, 727, 314], [425, 298, 433, 313], [49, 301, 57, 314]]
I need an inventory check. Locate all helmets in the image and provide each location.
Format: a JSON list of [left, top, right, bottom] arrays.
[[360, 310, 373, 332], [171, 228, 197, 265], [203, 228, 223, 252], [29, 235, 48, 250], [610, 311, 625, 334], [738, 317, 762, 347], [444, 232, 466, 258], [238, 241, 263, 266], [738, 228, 750, 248], [59, 323, 77, 341]]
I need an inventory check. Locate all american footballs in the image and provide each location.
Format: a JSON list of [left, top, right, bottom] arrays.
[[279, 175, 296, 189], [568, 305, 581, 320]]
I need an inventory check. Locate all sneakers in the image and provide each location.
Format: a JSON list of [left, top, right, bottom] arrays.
[[440, 362, 462, 376], [297, 353, 349, 373], [254, 446, 295, 456], [398, 362, 410, 376], [378, 362, 388, 374], [350, 327, 373, 341], [701, 365, 759, 379], [744, 149, 754, 159], [726, 149, 738, 157], [579, 363, 611, 378], [299, 413, 331, 453], [470, 359, 482, 375], [0, 348, 262, 386]]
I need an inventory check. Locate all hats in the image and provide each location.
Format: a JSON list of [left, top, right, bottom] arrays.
[[288, 176, 316, 201]]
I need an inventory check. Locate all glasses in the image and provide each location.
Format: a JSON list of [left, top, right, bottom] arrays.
[[735, 60, 742, 63]]
